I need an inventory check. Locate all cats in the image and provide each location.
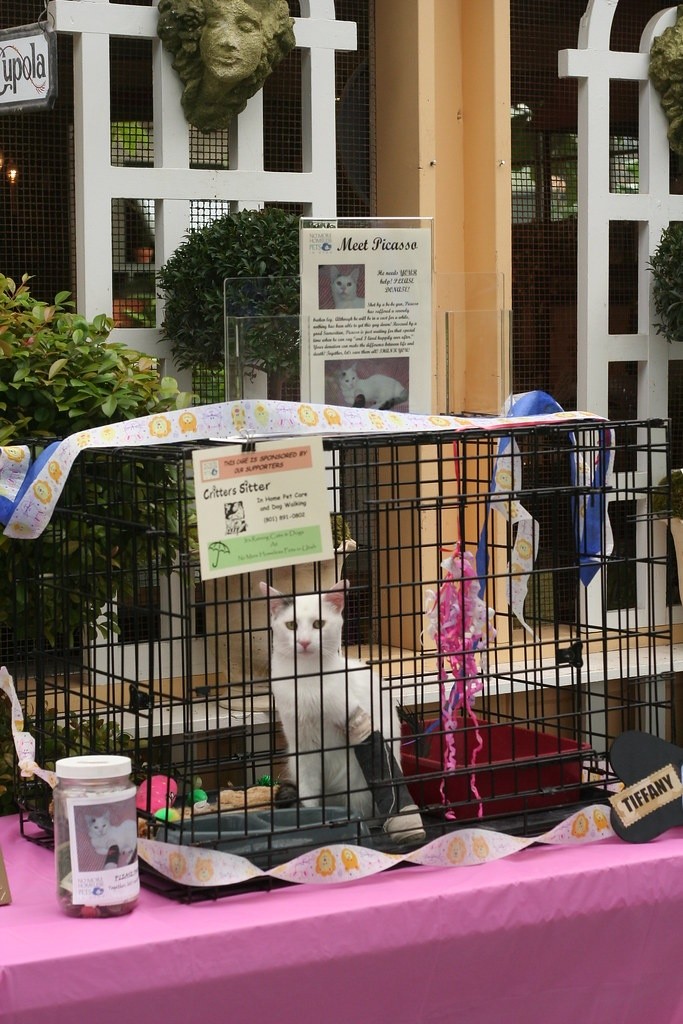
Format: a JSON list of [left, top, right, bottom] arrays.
[[338, 360, 409, 411], [327, 264, 365, 309], [257, 578, 403, 826]]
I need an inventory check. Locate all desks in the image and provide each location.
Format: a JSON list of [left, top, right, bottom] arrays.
[[0, 790, 682, 1024]]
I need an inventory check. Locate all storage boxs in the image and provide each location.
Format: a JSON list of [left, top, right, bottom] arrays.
[[403, 719, 591, 820]]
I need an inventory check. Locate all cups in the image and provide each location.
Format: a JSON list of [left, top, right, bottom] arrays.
[[52, 754, 141, 919]]
